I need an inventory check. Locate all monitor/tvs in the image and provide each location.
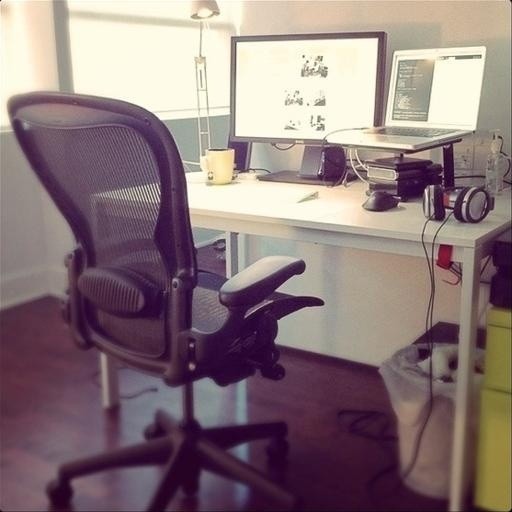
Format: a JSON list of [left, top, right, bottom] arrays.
[[228, 30, 388, 187]]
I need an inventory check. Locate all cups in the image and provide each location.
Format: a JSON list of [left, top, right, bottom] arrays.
[[201, 148, 234, 184]]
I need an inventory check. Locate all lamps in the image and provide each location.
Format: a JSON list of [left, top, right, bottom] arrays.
[[182, 1, 224, 168]]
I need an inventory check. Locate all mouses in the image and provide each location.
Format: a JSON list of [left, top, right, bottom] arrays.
[[362, 191, 399, 212]]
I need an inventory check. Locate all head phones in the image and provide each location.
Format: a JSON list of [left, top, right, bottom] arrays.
[[422, 184, 495, 224]]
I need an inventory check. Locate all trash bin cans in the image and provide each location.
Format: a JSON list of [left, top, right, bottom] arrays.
[[389, 342, 485, 498]]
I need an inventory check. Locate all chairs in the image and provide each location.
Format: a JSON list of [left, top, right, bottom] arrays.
[[5, 90, 329, 511]]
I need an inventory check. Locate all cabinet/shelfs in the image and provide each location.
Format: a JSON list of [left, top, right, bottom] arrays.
[[471, 297, 511, 510]]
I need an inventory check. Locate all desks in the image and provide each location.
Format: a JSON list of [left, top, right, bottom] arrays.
[[86, 169, 511, 510]]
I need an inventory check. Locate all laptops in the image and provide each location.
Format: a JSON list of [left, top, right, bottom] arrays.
[[324, 45, 487, 151]]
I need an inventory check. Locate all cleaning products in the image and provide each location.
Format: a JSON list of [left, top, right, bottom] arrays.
[[484, 129, 505, 196]]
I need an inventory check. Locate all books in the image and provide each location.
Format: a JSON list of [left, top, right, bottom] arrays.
[[367, 164, 443, 180], [366, 157, 433, 171]]
